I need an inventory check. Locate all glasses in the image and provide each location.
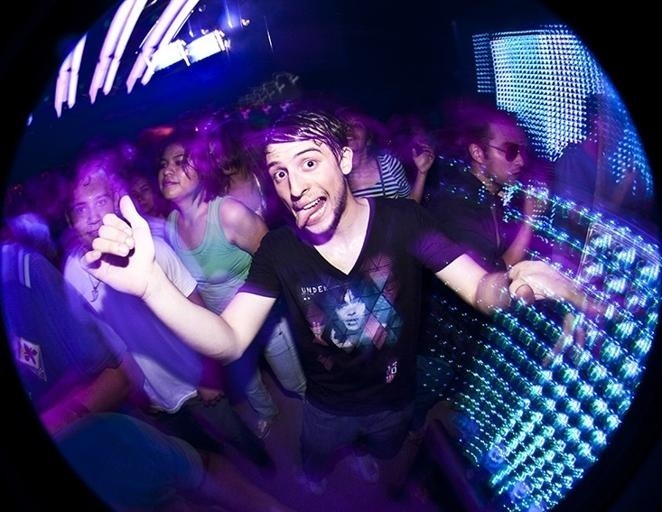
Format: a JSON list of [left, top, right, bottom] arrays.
[[484, 141, 524, 161]]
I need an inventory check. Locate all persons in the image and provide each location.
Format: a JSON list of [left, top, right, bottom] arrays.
[[4, 100, 606, 511], [552, 90, 640, 210]]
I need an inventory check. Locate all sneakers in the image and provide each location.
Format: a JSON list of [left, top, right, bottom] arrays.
[[253, 416, 274, 444]]
